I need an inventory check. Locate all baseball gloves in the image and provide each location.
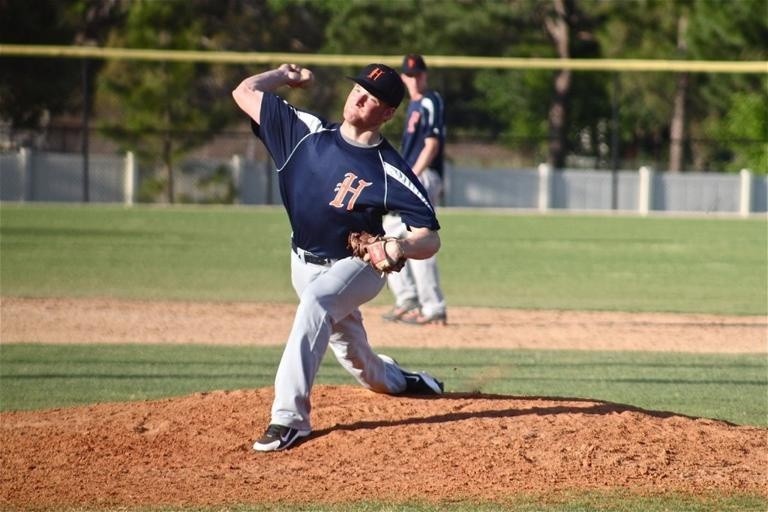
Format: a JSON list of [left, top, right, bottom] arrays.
[[348, 233, 406, 277]]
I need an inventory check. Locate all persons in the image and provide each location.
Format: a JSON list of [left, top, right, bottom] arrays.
[[381, 54, 446, 328], [231, 60, 444, 453]]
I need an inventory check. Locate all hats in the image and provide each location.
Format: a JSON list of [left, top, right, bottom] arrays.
[[400, 53, 428, 76], [344, 62, 407, 110]]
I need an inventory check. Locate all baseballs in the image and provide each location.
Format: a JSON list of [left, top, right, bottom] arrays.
[[301, 69, 313, 90]]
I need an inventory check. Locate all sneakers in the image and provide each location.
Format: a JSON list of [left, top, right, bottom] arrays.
[[379, 298, 447, 327], [376, 352, 446, 401], [251, 421, 312, 453]]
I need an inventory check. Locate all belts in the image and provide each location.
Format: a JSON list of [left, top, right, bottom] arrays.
[[290, 232, 330, 268]]
